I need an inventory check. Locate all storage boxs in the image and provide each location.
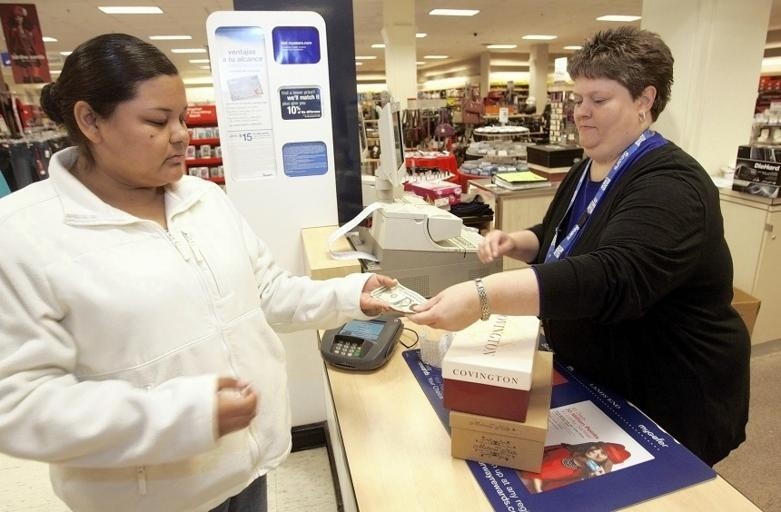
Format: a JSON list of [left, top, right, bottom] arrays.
[[442, 313, 556, 475], [526, 144, 584, 169]]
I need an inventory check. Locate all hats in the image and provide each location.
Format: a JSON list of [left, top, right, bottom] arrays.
[[600, 442, 630, 465]]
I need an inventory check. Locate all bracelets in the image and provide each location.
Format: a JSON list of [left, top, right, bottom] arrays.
[[475, 277, 491, 322]]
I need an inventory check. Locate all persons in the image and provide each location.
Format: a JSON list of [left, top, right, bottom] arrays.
[[403, 23, 751, 472], [517, 442, 630, 495], [2, 31, 398, 512]]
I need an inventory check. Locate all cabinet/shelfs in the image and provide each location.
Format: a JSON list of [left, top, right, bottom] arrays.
[[459, 107, 536, 193]]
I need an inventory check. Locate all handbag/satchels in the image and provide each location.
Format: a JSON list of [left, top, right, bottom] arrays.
[[403, 108, 455, 142], [461, 86, 486, 125]]
